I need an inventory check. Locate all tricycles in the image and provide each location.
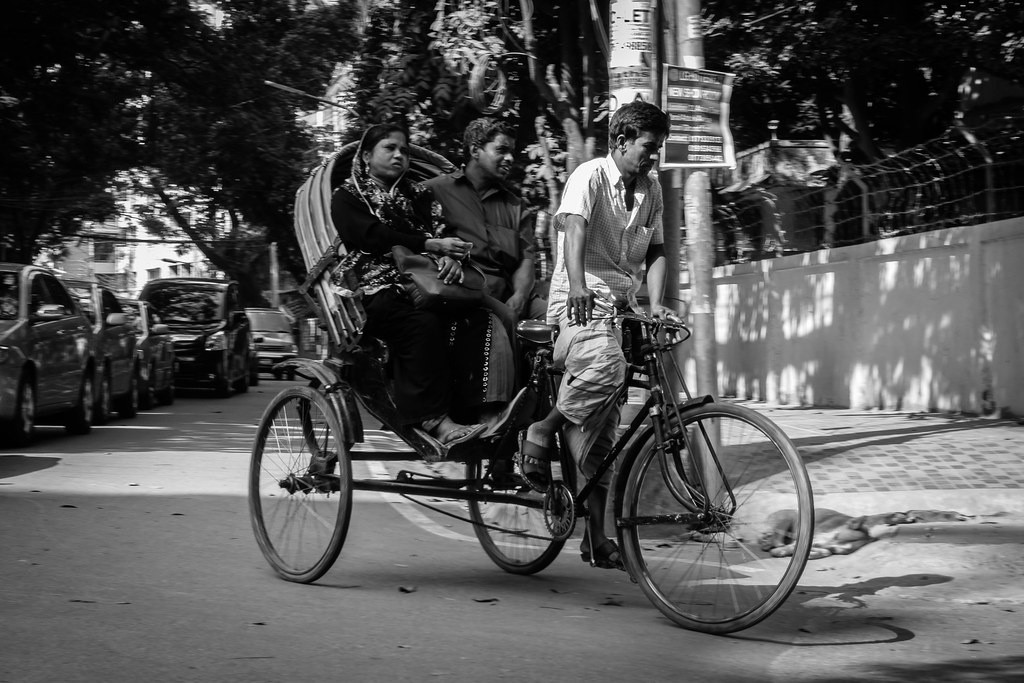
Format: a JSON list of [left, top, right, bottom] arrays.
[[249, 141, 815, 634]]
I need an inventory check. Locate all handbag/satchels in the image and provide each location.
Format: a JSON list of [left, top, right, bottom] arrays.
[[393, 245, 487, 313]]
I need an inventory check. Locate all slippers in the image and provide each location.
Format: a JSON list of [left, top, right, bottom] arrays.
[[580, 538, 649, 572], [444, 423, 488, 449], [518, 430, 553, 493]]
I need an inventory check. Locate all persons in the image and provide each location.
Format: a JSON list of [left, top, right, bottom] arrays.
[[518, 103, 681, 569], [331, 124, 488, 451], [411, 117, 552, 403]]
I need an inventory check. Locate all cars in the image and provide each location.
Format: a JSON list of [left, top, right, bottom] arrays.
[[119, 302, 175, 410], [66, 287, 138, 424], [0, 262, 96, 440]]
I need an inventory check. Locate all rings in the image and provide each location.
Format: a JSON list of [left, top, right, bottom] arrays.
[[457, 260, 462, 266]]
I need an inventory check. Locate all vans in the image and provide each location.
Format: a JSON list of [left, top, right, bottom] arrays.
[[245, 307, 299, 381]]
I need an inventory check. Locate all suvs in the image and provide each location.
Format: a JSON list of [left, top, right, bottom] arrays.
[[139, 277, 249, 396]]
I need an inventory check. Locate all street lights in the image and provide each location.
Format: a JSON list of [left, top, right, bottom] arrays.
[[264, 79, 364, 127]]
